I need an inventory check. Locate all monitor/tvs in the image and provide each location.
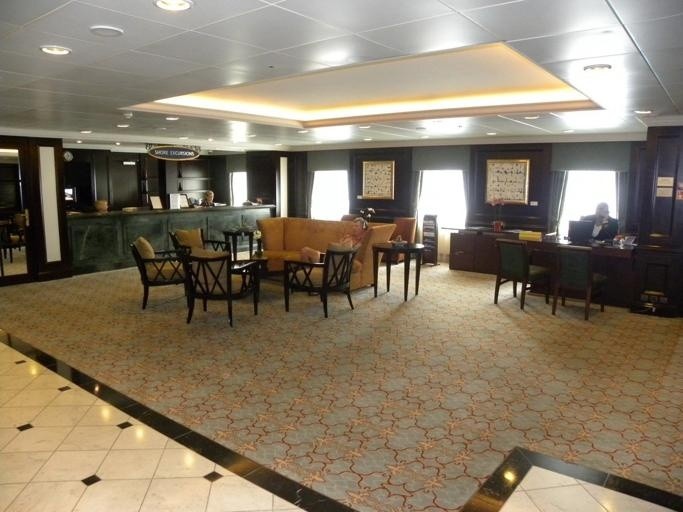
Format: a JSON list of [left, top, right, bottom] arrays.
[[567, 221, 596, 247], [63, 186, 74, 202]]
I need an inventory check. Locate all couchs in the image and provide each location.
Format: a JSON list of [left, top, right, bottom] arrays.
[[251, 216, 397, 292]]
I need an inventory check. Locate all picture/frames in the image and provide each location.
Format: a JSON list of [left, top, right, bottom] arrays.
[[485, 159, 529, 205], [362, 161, 394, 200]]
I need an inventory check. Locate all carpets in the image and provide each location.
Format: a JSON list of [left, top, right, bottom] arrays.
[[2, 266, 683, 512]]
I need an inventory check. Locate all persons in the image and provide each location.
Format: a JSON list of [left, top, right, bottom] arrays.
[[199, 190, 216, 206], [582, 201, 618, 241], [95, 200, 110, 211], [300, 217, 371, 264]]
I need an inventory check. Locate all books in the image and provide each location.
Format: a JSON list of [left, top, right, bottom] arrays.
[[518, 230, 543, 243]]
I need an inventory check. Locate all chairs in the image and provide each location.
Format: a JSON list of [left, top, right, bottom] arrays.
[[169, 228, 232, 262], [381, 217, 417, 265], [181, 251, 260, 328], [552, 243, 609, 320], [0, 214, 27, 263], [283, 249, 356, 318], [494, 239, 550, 310], [129, 236, 192, 310]]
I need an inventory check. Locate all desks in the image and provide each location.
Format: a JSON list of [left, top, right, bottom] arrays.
[[373, 242, 425, 302], [223, 225, 262, 261], [527, 230, 683, 309]]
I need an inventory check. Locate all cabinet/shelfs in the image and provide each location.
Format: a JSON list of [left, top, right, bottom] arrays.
[[449, 227, 546, 273]]
